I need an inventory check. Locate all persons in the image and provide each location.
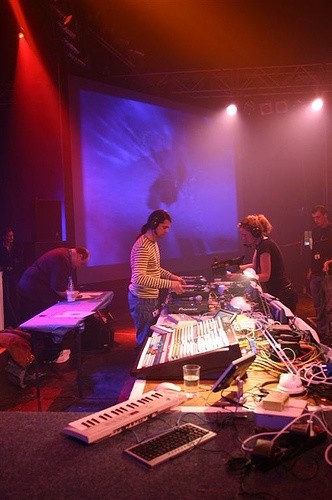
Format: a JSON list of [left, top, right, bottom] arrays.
[[308, 205, 332, 341], [16, 247, 89, 363], [226, 214, 298, 314], [128, 209, 186, 347], [0, 229, 23, 290]]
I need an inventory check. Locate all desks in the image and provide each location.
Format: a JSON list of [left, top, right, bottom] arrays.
[[0, 411, 332, 500], [20, 291, 115, 399], [117, 281, 332, 412]]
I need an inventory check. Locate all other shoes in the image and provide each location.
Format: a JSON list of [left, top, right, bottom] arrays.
[[42, 353, 69, 363], [60, 349, 71, 355]]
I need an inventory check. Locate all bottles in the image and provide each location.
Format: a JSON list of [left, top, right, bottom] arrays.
[[67, 276, 74, 291]]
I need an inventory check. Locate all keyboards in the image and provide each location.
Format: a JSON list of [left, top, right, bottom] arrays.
[[125, 423, 217, 467]]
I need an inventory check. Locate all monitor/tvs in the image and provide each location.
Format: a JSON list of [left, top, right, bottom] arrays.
[[209, 352, 256, 406]]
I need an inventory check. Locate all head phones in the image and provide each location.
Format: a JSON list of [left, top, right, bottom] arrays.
[[151, 210, 167, 225], [240, 221, 262, 238]]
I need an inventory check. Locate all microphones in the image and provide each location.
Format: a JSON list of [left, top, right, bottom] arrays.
[[176, 294, 202, 302]]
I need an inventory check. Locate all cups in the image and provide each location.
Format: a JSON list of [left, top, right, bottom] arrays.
[[183, 364, 201, 399]]
